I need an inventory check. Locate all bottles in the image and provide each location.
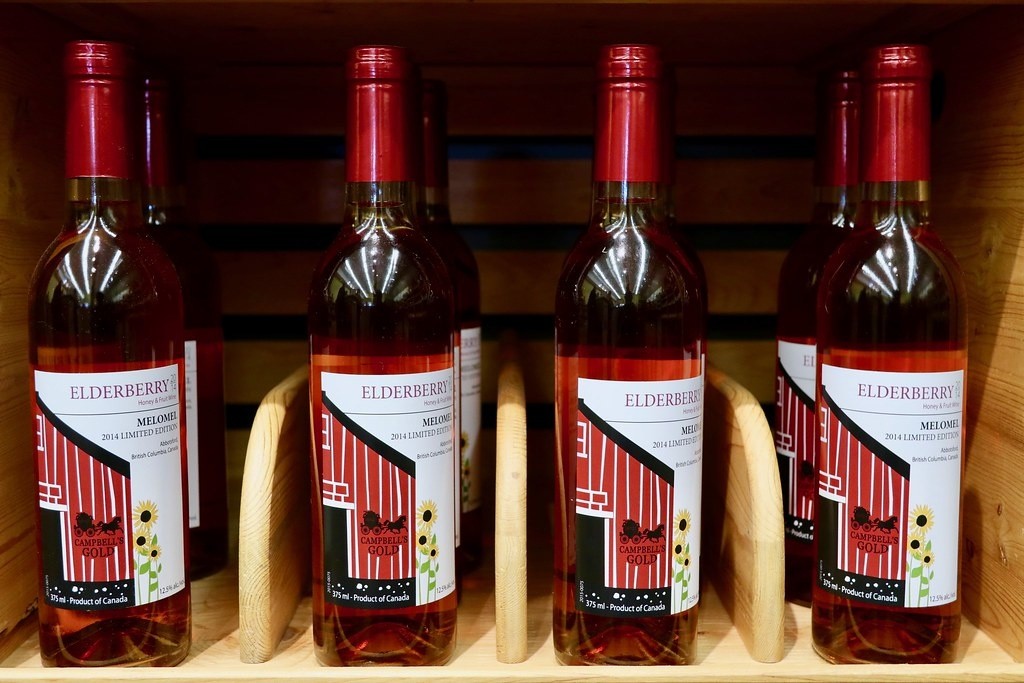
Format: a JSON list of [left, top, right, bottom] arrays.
[[812, 42, 970, 666], [766, 70, 867, 611], [415, 118, 491, 578], [553, 45, 708, 670], [625, 80, 705, 377], [309, 43, 457, 669], [127, 70, 230, 578], [25, 39, 193, 672]]
